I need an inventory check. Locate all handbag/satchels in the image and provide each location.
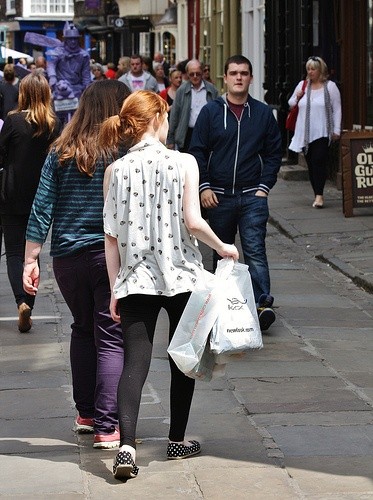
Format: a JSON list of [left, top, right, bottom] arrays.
[[285, 80, 307, 132], [166, 256, 235, 383], [210, 255, 264, 354]]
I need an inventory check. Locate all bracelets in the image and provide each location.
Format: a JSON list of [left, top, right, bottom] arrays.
[[22, 259, 37, 267]]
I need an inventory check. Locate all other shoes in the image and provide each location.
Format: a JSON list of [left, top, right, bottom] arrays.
[[312, 200, 323, 208], [257, 294, 276, 330], [93, 427, 120, 449], [113, 451, 139, 478], [18, 303, 33, 333], [74, 414, 95, 434], [166, 440, 201, 459]]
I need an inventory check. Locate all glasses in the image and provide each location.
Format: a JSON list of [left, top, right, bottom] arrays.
[[225, 70, 250, 77], [187, 72, 201, 77], [308, 56, 321, 65]]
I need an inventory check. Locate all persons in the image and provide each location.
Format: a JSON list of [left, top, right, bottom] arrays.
[[21, 79, 134, 449], [0, 70, 63, 333], [288, 55, 342, 207], [93, 89, 239, 478], [187, 53, 282, 331], [0, 21, 221, 225]]
